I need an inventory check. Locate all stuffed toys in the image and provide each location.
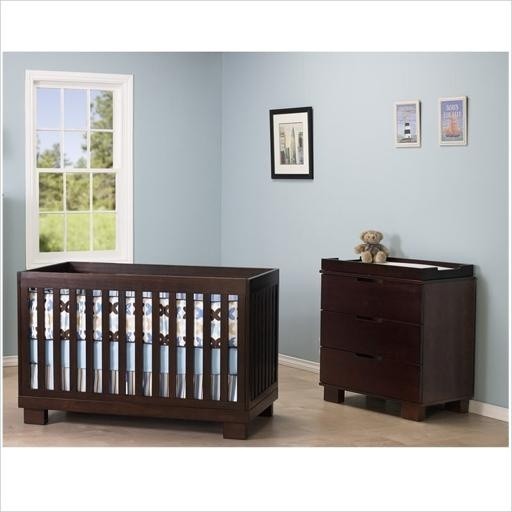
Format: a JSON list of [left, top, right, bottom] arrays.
[[355, 230, 390, 263]]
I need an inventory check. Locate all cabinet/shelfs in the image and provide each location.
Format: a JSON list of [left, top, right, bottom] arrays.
[[318, 256, 476, 419]]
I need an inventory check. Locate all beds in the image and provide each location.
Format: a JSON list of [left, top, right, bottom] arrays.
[[17, 261, 278, 438]]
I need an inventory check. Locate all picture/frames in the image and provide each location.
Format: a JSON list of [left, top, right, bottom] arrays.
[[392, 97, 421, 146], [437, 95, 469, 147], [268, 106, 314, 180]]
